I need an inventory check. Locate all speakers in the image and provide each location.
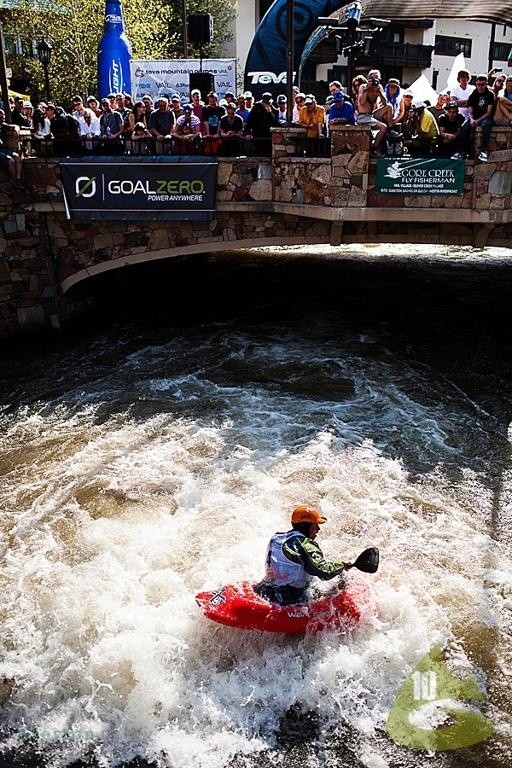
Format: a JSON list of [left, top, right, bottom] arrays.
[[189, 14, 214, 42], [189, 73, 215, 105]]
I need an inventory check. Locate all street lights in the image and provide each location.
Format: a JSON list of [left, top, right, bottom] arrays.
[[317, 16, 391, 97], [35, 36, 55, 102]]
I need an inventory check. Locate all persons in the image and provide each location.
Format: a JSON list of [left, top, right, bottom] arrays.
[[2, 65, 512, 190], [256, 503, 353, 608]]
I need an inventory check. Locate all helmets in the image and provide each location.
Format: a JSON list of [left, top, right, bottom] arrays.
[[291, 505, 327, 525]]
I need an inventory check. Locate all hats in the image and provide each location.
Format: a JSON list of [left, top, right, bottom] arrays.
[[304, 94, 315, 105], [389, 78, 400, 87], [171, 94, 180, 101], [277, 95, 287, 103], [444, 101, 458, 110], [332, 93, 343, 102], [411, 99, 427, 109]]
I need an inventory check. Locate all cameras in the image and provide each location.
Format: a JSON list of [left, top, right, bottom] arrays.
[[495, 69, 502, 72], [372, 79, 380, 86]]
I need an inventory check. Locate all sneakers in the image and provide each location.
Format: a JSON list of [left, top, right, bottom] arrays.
[[451, 153, 464, 161], [478, 152, 489, 162]]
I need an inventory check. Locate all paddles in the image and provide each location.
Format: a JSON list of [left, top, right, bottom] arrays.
[[344, 548, 379, 573]]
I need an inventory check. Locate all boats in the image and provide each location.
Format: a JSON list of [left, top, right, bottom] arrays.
[[193, 578, 365, 635]]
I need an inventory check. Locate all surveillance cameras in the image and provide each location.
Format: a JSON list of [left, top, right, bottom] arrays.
[[370, 18, 391, 27], [318, 16, 339, 25]]
